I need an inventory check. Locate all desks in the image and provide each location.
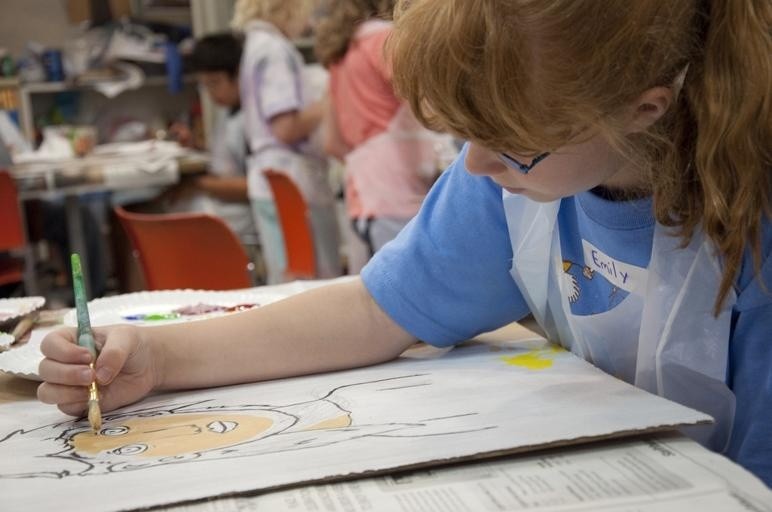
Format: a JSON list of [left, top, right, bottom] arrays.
[[20, 72, 201, 156], [14, 155, 207, 205]]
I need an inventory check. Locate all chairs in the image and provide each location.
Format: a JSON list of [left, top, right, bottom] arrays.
[[112, 205, 254, 291], [2, 174, 36, 299], [264, 168, 316, 284]]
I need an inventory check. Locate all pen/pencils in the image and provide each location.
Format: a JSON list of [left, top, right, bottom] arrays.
[[11, 310, 44, 345]]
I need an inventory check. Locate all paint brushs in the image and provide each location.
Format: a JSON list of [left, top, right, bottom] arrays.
[[72, 253, 103, 430]]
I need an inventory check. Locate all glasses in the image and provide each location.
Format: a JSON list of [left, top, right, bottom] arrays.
[[496, 133, 585, 175]]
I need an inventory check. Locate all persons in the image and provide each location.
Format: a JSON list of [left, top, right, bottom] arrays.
[[37, 2, 771, 488], [171, 29, 271, 287], [320, 1, 458, 259], [231, 0, 348, 287]]
